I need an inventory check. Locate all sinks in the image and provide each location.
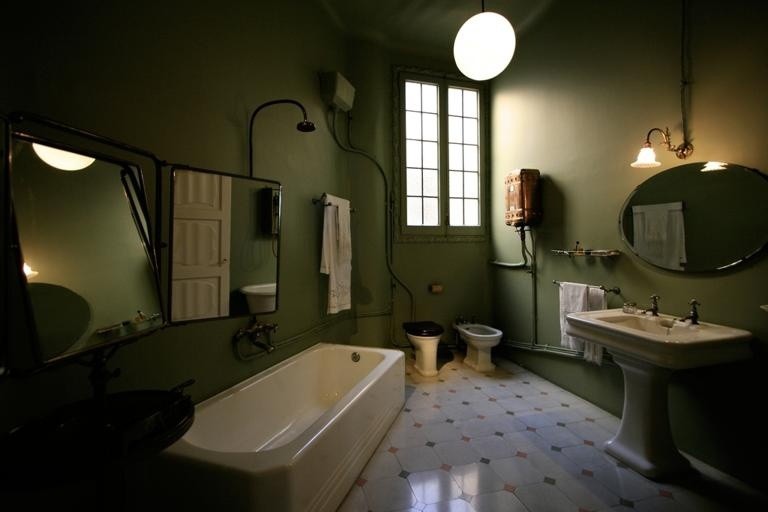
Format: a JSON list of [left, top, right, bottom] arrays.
[[241, 284, 276, 313], [566, 308, 751, 369]]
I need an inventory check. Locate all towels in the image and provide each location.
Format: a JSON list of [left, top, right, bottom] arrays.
[[644, 207, 669, 261], [585, 288, 607, 365], [558, 283, 588, 352], [338, 206, 355, 264]]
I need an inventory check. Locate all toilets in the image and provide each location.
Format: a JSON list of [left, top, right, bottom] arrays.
[[452, 315, 503, 372], [403, 321, 444, 377]]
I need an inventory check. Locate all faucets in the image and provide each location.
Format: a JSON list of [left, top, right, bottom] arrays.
[[679, 298, 701, 325], [641, 295, 660, 316]]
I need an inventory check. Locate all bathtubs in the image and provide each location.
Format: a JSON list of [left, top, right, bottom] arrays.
[[164, 343, 405, 512]]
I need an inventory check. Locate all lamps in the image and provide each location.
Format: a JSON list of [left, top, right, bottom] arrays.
[[628, 0, 695, 170], [248, 97, 316, 176]]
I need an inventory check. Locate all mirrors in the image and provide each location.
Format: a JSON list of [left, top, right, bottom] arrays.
[[0, 109, 169, 378], [167, 163, 283, 328], [615, 160, 768, 278]]
